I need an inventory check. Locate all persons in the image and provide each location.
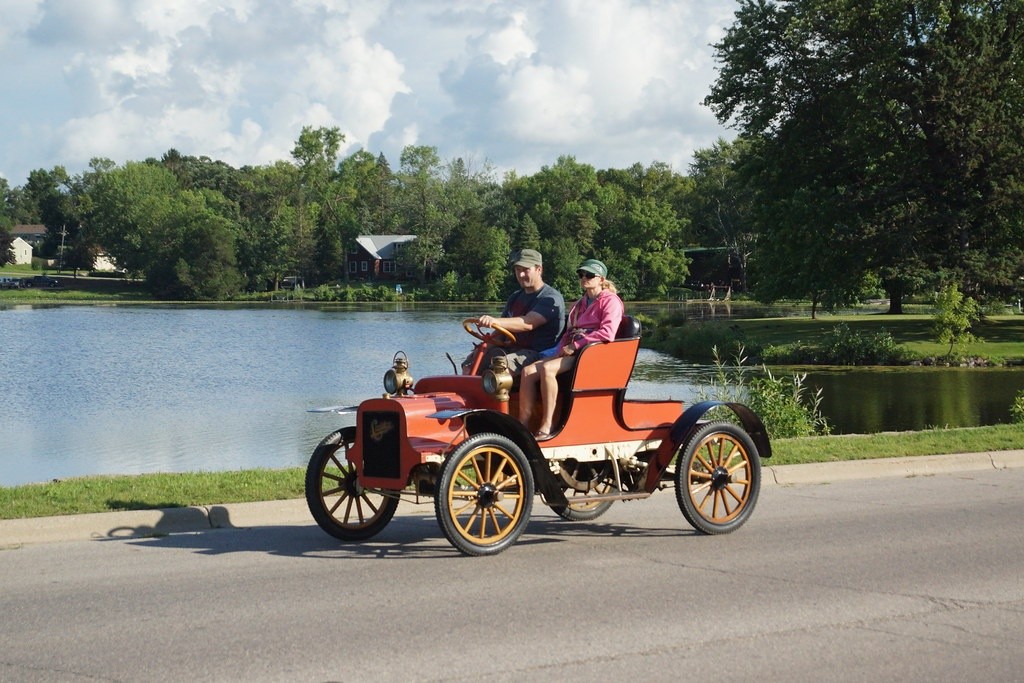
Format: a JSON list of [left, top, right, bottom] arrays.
[[518, 259, 624, 441], [461, 249, 566, 377]]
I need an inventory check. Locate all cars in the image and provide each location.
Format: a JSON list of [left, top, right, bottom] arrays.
[[265, 275, 302, 290], [305, 311, 773, 556]]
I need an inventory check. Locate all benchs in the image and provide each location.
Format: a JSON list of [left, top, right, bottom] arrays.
[[463, 312, 642, 393]]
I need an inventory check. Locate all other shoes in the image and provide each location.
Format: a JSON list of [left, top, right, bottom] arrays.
[[530, 430, 549, 439]]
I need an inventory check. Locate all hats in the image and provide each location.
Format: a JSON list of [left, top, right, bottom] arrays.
[[511, 249, 542, 268], [576, 259, 608, 277]]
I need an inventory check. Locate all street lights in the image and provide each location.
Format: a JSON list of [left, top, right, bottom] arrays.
[[58, 224, 70, 275]]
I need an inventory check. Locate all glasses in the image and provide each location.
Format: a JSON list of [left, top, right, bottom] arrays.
[[579, 272, 600, 279]]
